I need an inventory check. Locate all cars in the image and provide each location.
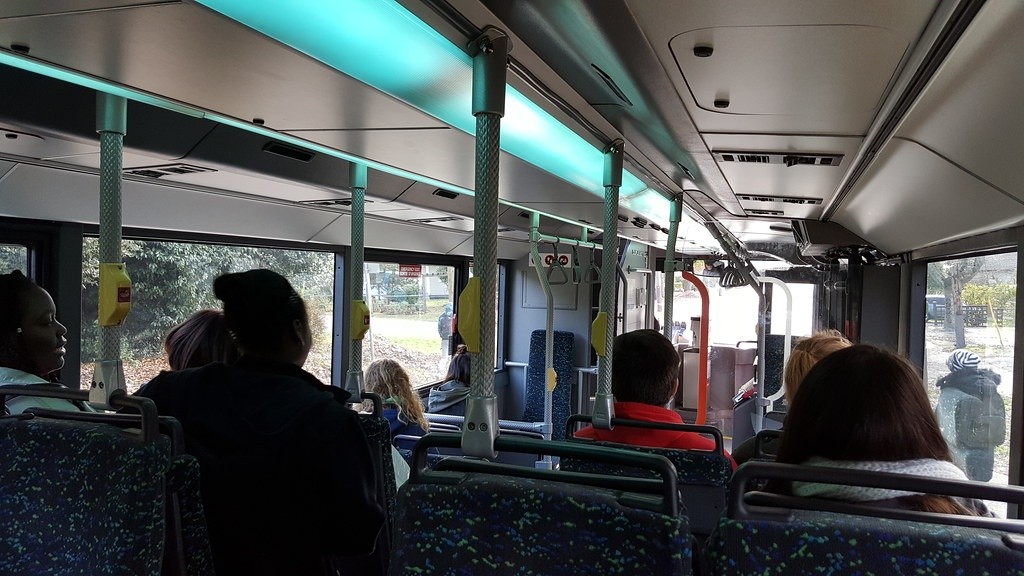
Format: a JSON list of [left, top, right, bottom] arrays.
[[388, 287, 414, 302], [925, 295, 987, 325]]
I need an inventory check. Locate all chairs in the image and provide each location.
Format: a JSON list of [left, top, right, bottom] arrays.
[[0, 384, 1024, 576]]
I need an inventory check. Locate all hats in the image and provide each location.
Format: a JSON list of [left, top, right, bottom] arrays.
[[946, 350, 980, 373]]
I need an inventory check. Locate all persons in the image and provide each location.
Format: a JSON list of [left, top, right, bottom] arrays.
[[427, 344, 471, 415], [354, 359, 440, 454], [123, 269, 385, 576], [0, 270, 79, 414], [785, 333, 856, 412], [576, 329, 737, 472], [165, 311, 238, 371], [756, 345, 993, 518], [438, 302, 452, 356], [935, 349, 1005, 481]]
